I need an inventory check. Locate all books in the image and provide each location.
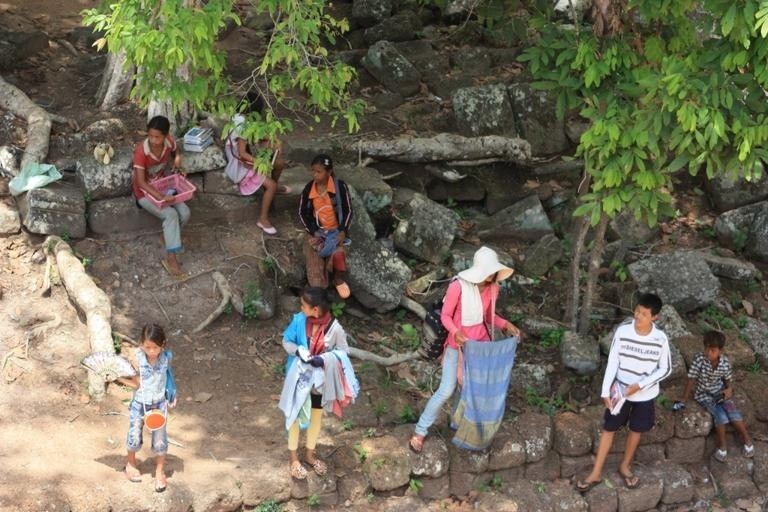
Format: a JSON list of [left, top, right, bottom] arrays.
[[609, 380, 626, 416], [183, 126, 215, 153]]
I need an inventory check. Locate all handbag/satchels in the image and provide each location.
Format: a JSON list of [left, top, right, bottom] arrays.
[[144, 409, 166, 431], [317, 228, 339, 257], [418, 311, 448, 360]]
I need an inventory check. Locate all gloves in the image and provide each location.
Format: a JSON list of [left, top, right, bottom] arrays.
[[295, 350, 311, 363], [312, 357, 323, 366]]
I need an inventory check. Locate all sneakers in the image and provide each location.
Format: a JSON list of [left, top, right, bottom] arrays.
[[276, 184, 291, 194], [256, 222, 277, 234]]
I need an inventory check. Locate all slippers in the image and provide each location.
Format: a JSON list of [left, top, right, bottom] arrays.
[[126, 464, 141, 481], [410, 433, 424, 453], [333, 279, 351, 299], [155, 472, 166, 492], [162, 260, 180, 273], [576, 478, 603, 492], [715, 447, 728, 462], [290, 461, 306, 479], [313, 459, 326, 476], [158, 234, 185, 255], [618, 467, 639, 488], [743, 443, 754, 457]]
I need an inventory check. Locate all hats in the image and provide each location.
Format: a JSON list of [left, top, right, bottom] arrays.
[[458, 247, 514, 283]]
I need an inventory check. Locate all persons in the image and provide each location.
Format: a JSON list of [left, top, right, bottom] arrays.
[[108, 323, 177, 493], [409, 246, 521, 454], [224, 91, 292, 235], [133, 115, 191, 278], [671, 330, 755, 463], [297, 154, 352, 299], [575, 292, 672, 493], [277, 287, 350, 480]]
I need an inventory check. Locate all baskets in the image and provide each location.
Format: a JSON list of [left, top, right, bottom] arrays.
[[139, 174, 197, 209]]
